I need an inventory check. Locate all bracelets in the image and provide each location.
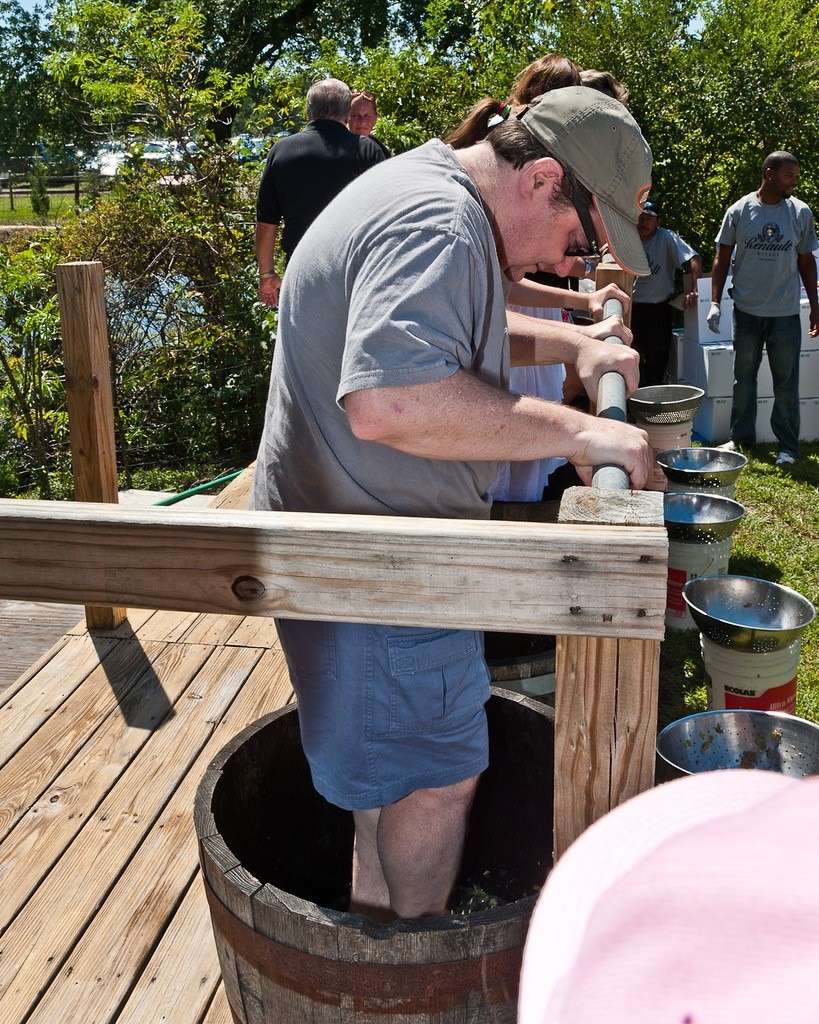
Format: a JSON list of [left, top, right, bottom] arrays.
[[248, 51, 702, 921]]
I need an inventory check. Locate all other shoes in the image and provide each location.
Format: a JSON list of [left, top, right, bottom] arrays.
[[774, 450, 799, 469], [719, 439, 751, 460]]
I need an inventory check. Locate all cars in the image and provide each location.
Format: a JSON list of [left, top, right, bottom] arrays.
[[8, 133, 266, 176]]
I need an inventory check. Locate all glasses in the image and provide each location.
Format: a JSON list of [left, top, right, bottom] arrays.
[[566, 177, 601, 262], [347, 90, 378, 101]]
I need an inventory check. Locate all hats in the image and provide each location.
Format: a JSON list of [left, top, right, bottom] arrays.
[[520, 85, 656, 280], [643, 203, 658, 219]]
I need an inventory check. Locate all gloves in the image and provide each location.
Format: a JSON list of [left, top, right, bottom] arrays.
[[707, 300, 723, 334]]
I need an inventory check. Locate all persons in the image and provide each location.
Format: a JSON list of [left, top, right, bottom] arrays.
[[706, 151, 819, 470]]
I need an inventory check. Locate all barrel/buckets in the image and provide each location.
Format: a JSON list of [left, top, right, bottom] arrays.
[[665, 538, 733, 630], [698, 632, 800, 717], [662, 482, 737, 501], [636, 421, 696, 492]]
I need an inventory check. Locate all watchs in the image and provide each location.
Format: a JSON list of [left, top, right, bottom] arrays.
[[259, 270, 275, 279], [579, 257, 592, 280]]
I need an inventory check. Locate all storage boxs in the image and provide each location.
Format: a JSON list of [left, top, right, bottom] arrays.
[[684, 273, 733, 344], [678, 337, 697, 385], [696, 343, 733, 398], [756, 398, 779, 443], [695, 397, 732, 443], [798, 398, 819, 442], [798, 351, 819, 399], [799, 299, 819, 351], [756, 350, 774, 398]]
